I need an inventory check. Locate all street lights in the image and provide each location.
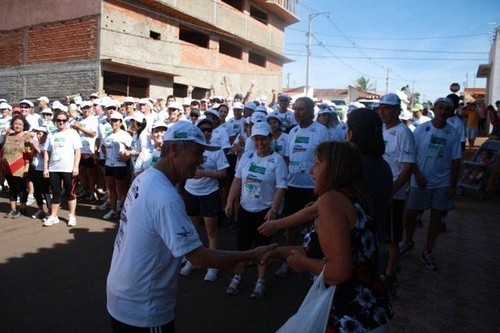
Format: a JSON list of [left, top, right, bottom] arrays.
[[306, 11, 331, 95]]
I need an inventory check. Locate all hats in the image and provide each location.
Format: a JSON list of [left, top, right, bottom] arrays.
[[0, 92, 462, 146], [108, 111, 123, 124], [161, 119, 214, 151]]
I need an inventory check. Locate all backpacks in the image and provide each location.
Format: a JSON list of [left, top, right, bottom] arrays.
[[359, 240, 397, 297]]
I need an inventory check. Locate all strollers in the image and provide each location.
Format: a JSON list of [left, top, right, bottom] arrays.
[[455, 137, 500, 201]]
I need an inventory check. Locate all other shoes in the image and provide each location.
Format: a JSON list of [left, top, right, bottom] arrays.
[[100, 194, 108, 200], [398, 239, 415, 255], [250, 278, 268, 300], [421, 250, 435, 270], [31, 209, 44, 219], [203, 268, 219, 282], [179, 260, 195, 276], [97, 201, 112, 211], [67, 214, 77, 227], [102, 210, 119, 219], [226, 273, 243, 296], [4, 208, 17, 218], [43, 215, 60, 226]]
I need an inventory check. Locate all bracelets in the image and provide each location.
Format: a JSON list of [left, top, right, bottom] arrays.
[[271, 207, 278, 212]]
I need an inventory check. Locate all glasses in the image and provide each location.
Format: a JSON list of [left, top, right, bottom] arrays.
[[57, 118, 68, 123]]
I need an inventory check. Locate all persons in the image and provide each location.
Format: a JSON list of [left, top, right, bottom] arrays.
[[104, 119, 277, 333], [257, 108, 399, 277], [0, 93, 500, 303], [255, 141, 394, 333]]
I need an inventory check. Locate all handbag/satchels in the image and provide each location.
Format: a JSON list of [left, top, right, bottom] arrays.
[[273, 265, 337, 333]]
